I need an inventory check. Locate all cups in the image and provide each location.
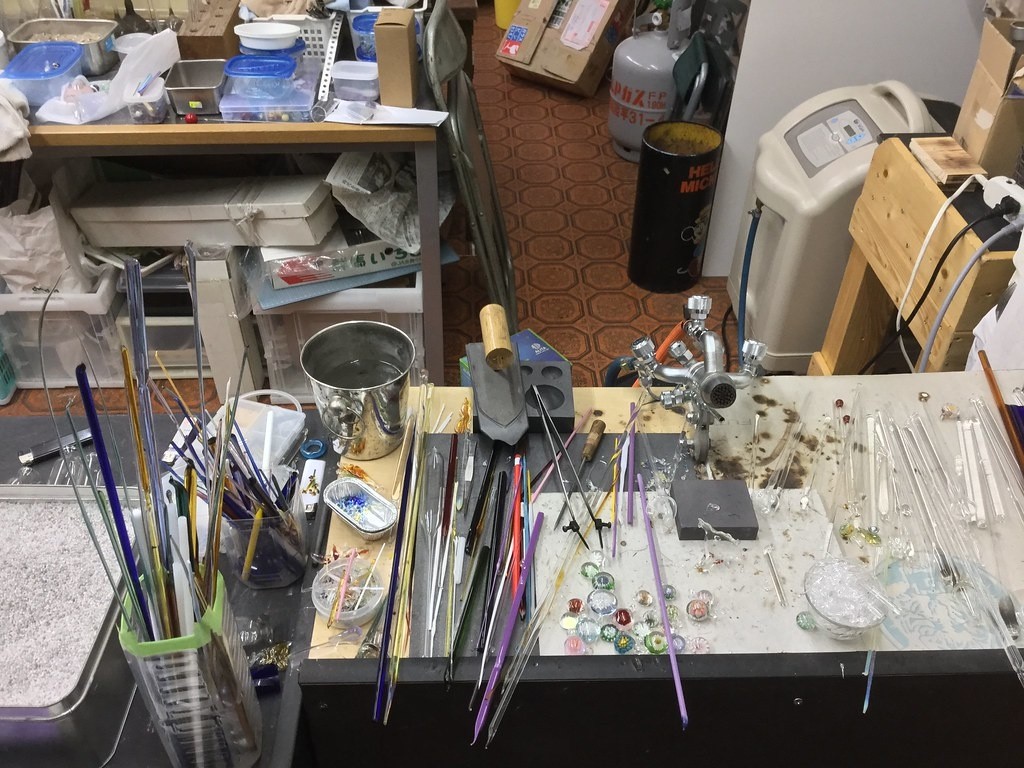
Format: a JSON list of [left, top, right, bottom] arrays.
[[219, 466, 309, 589], [116, 561, 262, 768]]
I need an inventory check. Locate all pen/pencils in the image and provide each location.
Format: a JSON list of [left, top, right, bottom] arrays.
[[478, 470, 509, 652], [312, 505, 330, 568], [464, 442, 500, 555], [512, 453, 521, 598], [242, 508, 263, 582], [519, 497, 527, 620]]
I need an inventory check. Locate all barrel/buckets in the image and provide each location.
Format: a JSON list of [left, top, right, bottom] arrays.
[[299, 319, 415, 461]]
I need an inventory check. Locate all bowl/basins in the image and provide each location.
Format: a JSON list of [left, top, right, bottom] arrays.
[[805, 560, 886, 639], [233, 22, 301, 50], [311, 558, 383, 627]]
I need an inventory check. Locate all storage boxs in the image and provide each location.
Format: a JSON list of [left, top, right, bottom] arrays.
[[70, 172, 339, 246], [250, 271, 424, 406], [373, 8, 420, 108], [238, 39, 307, 65], [330, 61, 379, 102], [952, 15, 1024, 188], [173, 396, 306, 467], [495, 0, 635, 99], [219, 57, 323, 123], [260, 223, 421, 292], [115, 249, 193, 317], [0, 40, 84, 107], [458, 328, 572, 387], [116, 301, 214, 381], [223, 54, 297, 102], [187, 247, 264, 405], [352, 14, 420, 56], [0, 266, 125, 390]]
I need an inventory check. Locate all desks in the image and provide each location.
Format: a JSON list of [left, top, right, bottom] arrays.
[[0, 409, 341, 768], [297, 368, 1024, 768], [26, 60, 445, 387]]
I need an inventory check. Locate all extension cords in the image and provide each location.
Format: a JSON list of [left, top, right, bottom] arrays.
[[983, 176, 1024, 232]]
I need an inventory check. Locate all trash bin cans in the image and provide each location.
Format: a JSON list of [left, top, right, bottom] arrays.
[[627, 121, 724, 293]]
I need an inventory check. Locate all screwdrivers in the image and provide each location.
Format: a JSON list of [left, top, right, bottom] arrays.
[[552, 419, 607, 531]]
